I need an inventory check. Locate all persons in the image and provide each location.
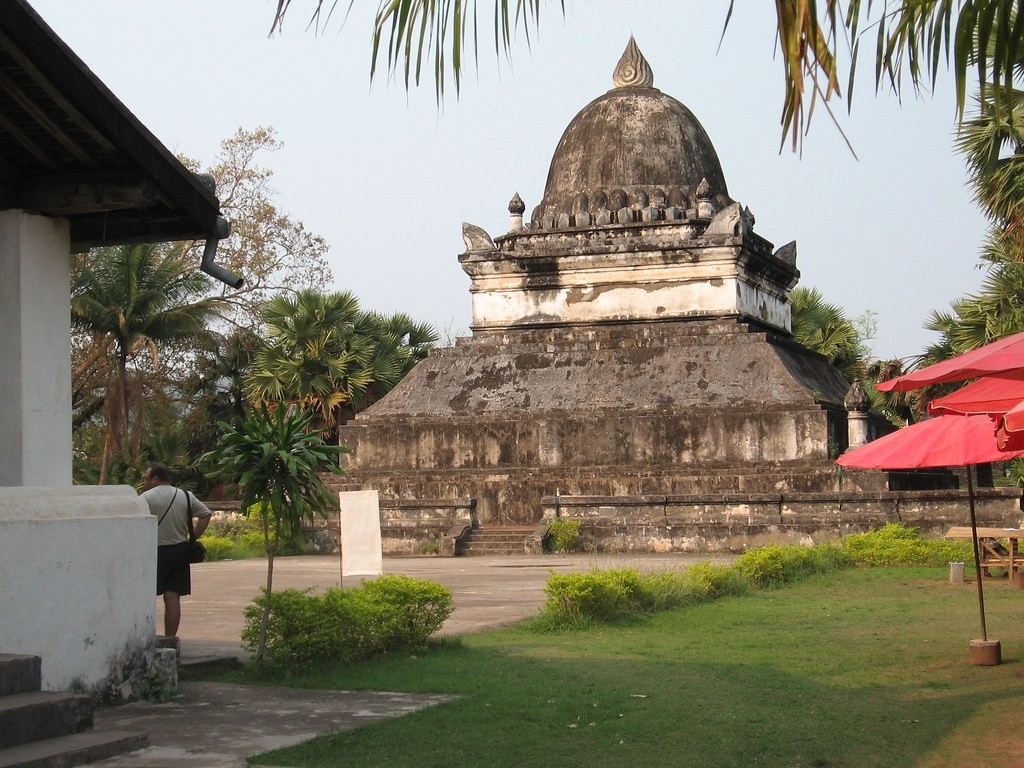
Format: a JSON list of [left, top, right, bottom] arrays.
[[139, 463, 212, 636]]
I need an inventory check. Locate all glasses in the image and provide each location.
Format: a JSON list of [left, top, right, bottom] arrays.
[[142, 476, 150, 482]]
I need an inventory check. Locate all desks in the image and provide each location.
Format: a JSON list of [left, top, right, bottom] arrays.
[[945, 526, 1024, 582]]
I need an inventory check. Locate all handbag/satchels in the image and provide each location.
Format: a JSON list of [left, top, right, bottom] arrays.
[[183, 489, 207, 563]]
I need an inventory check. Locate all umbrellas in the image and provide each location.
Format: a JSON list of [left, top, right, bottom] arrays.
[[927, 367, 1024, 418], [995, 400, 1024, 452], [837, 415, 1024, 641], [875, 331, 1024, 393]]
